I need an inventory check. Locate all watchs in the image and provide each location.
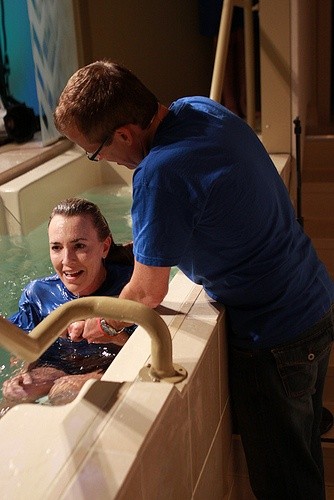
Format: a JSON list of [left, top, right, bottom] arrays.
[[101, 318, 125, 336]]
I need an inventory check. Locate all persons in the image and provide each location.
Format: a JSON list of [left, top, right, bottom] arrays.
[[0, 198, 138, 419], [54, 61, 334, 500]]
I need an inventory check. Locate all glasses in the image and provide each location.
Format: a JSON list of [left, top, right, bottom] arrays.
[[84, 133, 113, 161]]
